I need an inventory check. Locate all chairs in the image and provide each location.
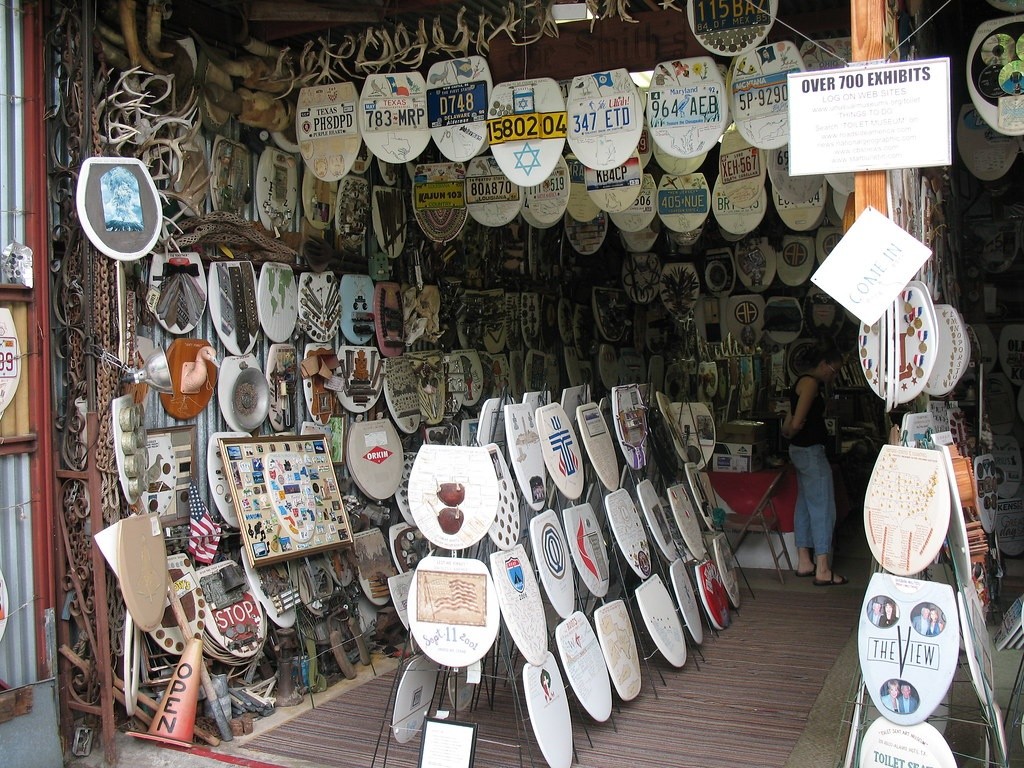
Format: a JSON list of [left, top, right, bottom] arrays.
[[717, 464, 795, 584]]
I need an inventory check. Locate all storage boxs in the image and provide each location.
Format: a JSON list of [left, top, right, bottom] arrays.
[[713, 439, 766, 472]]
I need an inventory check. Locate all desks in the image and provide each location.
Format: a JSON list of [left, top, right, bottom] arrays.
[[708, 468, 796, 571]]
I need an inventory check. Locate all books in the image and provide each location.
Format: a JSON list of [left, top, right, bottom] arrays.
[[834, 361, 866, 388]]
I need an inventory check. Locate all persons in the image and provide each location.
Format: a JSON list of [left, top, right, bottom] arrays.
[[869, 600, 943, 715], [780, 346, 849, 586]]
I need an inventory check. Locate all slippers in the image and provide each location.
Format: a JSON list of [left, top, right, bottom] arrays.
[[795, 564, 817, 577], [813, 570, 849, 585]]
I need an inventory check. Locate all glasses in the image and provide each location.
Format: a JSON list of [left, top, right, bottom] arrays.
[[827, 364, 838, 376]]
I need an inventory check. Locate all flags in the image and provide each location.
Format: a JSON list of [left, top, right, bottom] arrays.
[[186, 482, 220, 564]]
